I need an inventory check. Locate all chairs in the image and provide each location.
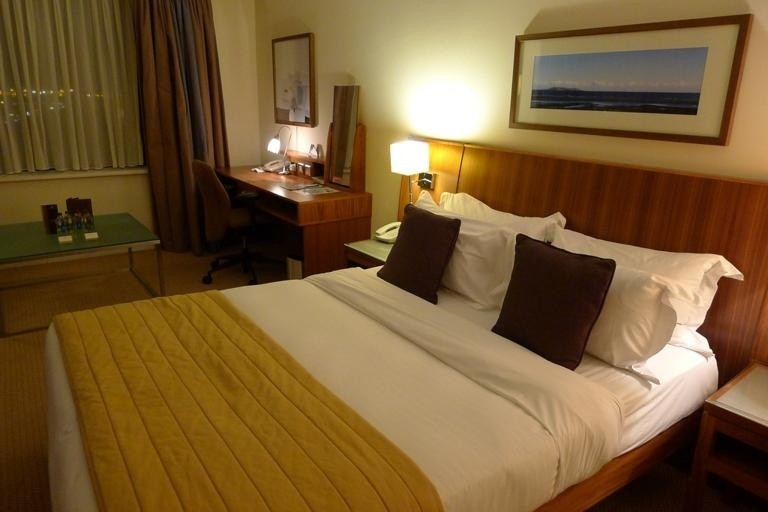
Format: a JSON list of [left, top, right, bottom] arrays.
[[190, 157, 279, 286]]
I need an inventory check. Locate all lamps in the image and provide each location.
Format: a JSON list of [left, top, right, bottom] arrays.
[[389, 137, 431, 205], [266, 125, 294, 176]]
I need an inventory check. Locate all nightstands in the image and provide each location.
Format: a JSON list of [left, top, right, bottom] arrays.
[[686, 357, 767, 511]]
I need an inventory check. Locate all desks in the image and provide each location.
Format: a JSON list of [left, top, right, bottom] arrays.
[[213, 161, 372, 277], [1, 209, 166, 333], [341, 237, 392, 269]]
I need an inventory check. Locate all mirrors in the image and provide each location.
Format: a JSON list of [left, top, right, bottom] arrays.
[[324, 84, 367, 194]]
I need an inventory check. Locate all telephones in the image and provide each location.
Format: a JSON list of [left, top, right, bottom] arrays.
[[376, 221, 402, 243], [264, 160, 290, 173]]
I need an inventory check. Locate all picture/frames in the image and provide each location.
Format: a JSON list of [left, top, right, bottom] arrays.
[[270, 31, 315, 130], [507, 9, 757, 147]]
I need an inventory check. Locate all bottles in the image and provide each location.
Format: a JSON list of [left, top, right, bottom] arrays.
[[54, 208, 95, 236]]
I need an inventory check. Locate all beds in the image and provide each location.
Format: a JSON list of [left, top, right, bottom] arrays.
[[46, 130, 768, 512]]
